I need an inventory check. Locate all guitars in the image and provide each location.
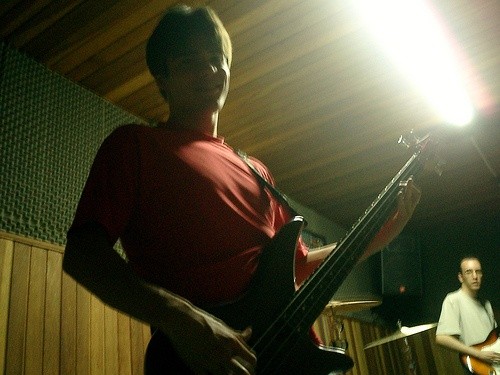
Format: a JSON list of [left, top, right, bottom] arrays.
[[144, 131, 443, 375], [459, 328, 500, 375]]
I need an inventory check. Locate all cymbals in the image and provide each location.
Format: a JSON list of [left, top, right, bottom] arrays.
[[362, 323, 437, 351]]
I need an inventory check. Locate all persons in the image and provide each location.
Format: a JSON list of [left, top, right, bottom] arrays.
[[61, 3, 423, 375], [432, 256, 500, 375]]
